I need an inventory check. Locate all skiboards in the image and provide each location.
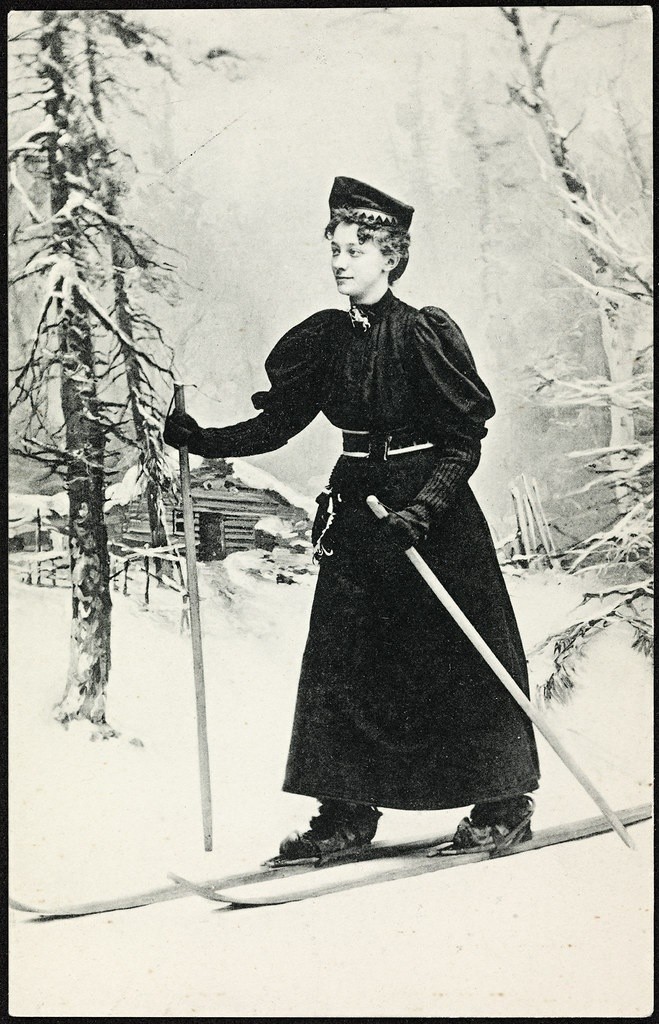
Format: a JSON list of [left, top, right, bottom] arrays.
[[7, 804, 656, 920]]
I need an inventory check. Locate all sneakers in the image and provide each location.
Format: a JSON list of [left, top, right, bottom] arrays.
[[456, 796, 533, 847], [280, 803, 382, 858]]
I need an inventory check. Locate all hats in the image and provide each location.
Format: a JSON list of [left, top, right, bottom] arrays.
[[329, 177, 414, 233]]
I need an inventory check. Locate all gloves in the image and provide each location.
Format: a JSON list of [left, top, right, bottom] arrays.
[[381, 511, 421, 552], [164, 408, 201, 454]]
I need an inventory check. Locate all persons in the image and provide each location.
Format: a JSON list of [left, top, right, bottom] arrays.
[[162, 175, 543, 862]]
